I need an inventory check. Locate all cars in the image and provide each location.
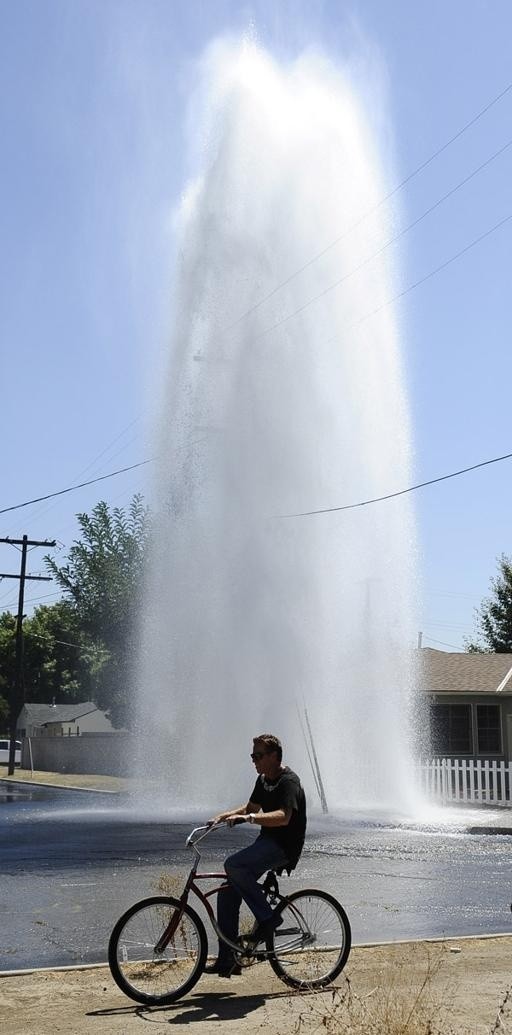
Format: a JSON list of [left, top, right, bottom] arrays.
[[0, 740, 24, 767]]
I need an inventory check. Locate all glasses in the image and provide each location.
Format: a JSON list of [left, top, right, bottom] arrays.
[[251, 751, 267, 760]]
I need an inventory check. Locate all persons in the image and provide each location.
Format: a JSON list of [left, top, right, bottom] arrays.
[[204, 735, 307, 975]]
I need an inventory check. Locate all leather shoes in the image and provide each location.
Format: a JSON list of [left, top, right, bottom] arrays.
[[241, 913, 283, 942], [203, 958, 241, 975]]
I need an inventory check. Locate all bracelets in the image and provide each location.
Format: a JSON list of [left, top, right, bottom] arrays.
[[250, 813, 256, 824]]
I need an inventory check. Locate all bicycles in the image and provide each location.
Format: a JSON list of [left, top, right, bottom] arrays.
[[105, 817, 353, 1006]]
